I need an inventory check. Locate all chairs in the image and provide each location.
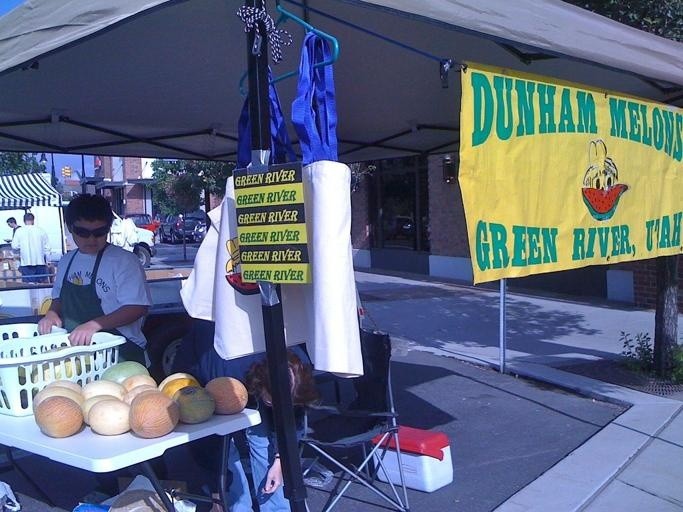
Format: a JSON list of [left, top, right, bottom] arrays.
[[291, 326, 411, 512]]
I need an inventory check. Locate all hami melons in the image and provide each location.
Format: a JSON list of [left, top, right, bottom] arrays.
[[31, 361, 249, 439]]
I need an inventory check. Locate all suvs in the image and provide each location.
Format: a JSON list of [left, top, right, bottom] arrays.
[[127, 214, 154, 232], [158, 212, 204, 241], [106, 212, 157, 269]]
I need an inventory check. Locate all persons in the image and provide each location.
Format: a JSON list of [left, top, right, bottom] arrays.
[[171, 344, 322, 511], [37, 193, 152, 377], [11, 213, 52, 281], [3, 217, 20, 243]]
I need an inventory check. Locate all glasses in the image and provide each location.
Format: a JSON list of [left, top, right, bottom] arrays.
[[72, 224, 109, 239]]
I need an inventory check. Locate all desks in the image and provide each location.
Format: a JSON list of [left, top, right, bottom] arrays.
[[0, 279, 192, 323], [0, 407, 262, 510]]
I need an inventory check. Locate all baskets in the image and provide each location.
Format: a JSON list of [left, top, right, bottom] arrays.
[[0, 331, 127, 417], [0, 323, 67, 336]]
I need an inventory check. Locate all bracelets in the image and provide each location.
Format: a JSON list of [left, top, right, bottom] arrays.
[[272, 454, 280, 460]]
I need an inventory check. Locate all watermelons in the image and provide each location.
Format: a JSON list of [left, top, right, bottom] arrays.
[[582, 183, 629, 221]]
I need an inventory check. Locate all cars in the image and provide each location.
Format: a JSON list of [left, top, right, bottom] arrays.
[[194, 223, 205, 241]]
